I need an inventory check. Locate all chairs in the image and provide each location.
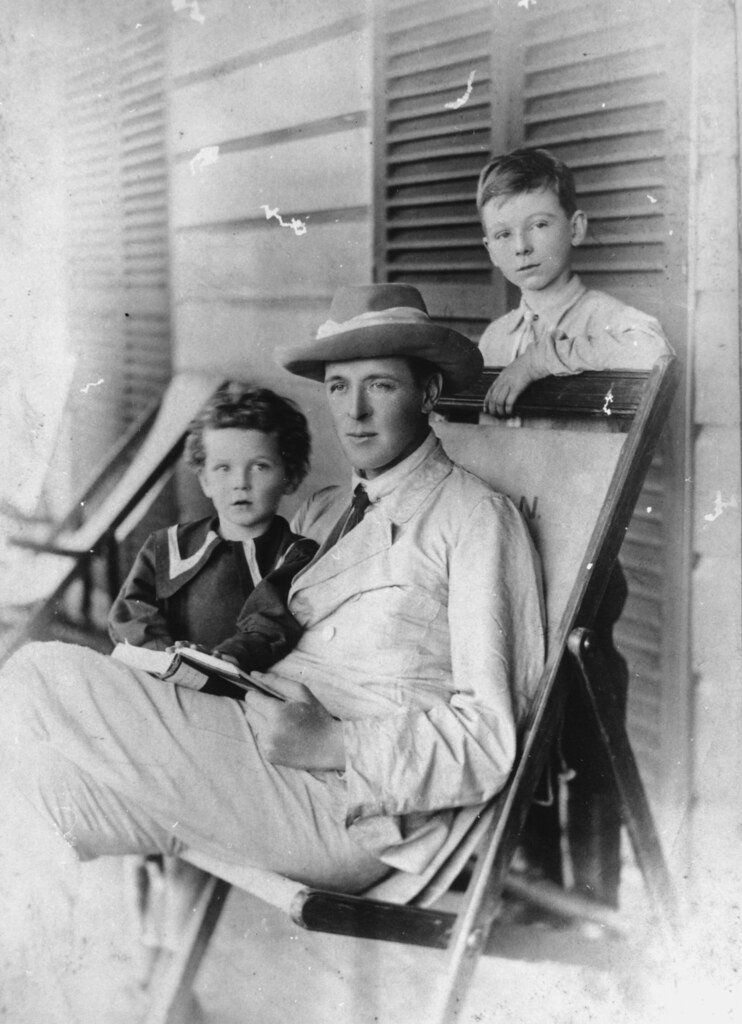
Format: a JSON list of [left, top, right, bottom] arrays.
[[0, 365, 242, 670], [124, 355, 683, 1024]]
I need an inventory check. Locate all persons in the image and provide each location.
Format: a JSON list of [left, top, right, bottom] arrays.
[[2, 152, 680, 892]]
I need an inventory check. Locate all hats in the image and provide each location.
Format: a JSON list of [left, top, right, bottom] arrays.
[[282, 283, 485, 397]]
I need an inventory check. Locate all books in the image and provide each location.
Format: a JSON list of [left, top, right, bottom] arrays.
[[111, 640, 286, 702]]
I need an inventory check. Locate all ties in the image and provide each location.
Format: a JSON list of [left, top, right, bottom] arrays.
[[511, 311, 537, 362], [300, 488, 369, 580]]
